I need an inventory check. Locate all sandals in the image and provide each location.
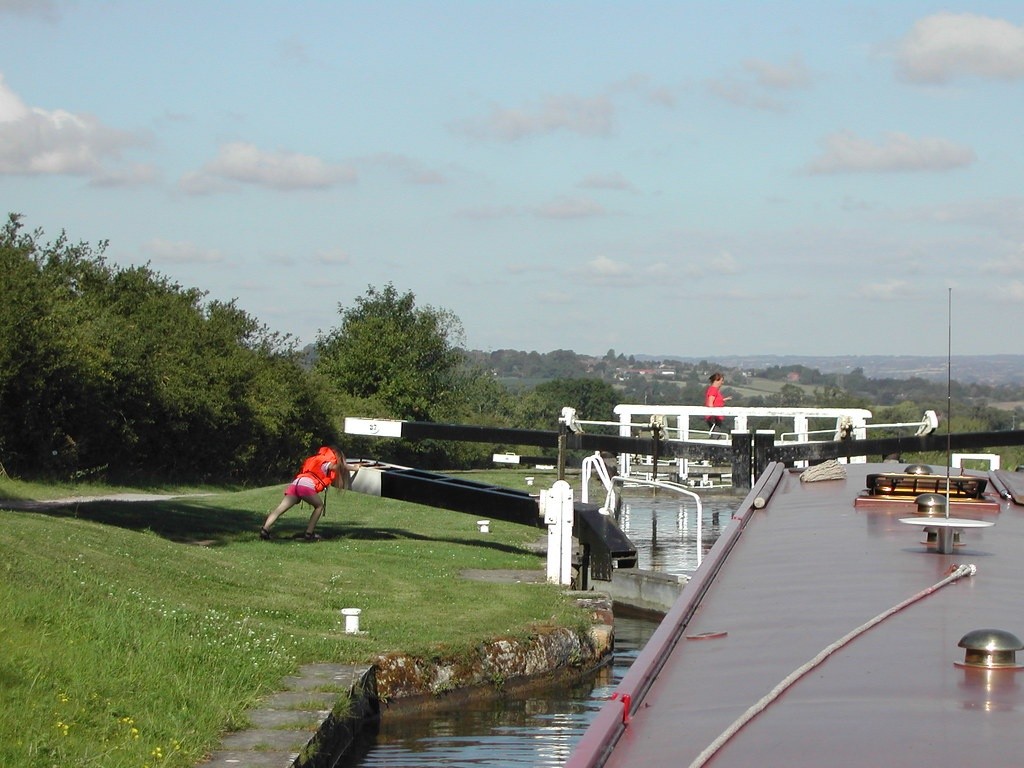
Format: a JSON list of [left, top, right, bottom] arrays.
[[261, 527, 272, 541], [304, 532, 320, 540]]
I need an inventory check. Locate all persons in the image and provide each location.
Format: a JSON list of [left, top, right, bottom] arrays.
[[698, 373, 733, 467], [259, 448, 361, 542]]
